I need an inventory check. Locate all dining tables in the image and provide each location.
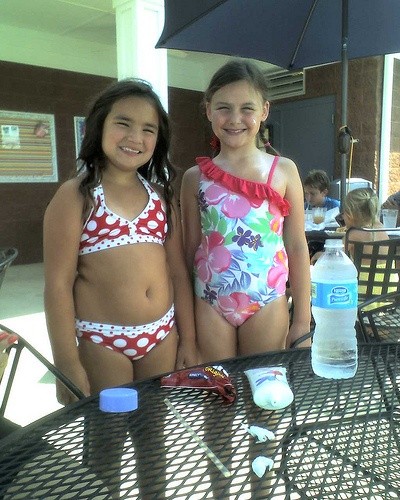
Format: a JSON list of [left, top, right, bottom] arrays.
[[304, 226, 400, 245]]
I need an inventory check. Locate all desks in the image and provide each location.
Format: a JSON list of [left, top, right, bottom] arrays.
[[0, 341, 400, 500]]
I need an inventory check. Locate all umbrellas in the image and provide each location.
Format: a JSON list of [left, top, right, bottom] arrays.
[[156, 0, 400, 213]]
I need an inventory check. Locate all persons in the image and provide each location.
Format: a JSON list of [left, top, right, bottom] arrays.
[[312, 188, 400, 312], [303, 168, 340, 212], [179, 60, 313, 500], [0, 333, 18, 380], [43, 78, 198, 500]]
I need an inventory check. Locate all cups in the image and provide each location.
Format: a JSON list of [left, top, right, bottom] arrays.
[[382, 208, 399, 229], [312, 206, 328, 225]]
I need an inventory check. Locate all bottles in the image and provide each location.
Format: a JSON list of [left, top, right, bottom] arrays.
[[309, 235, 362, 380]]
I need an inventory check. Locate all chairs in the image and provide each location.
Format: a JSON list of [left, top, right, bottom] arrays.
[[0, 227, 400, 500]]
